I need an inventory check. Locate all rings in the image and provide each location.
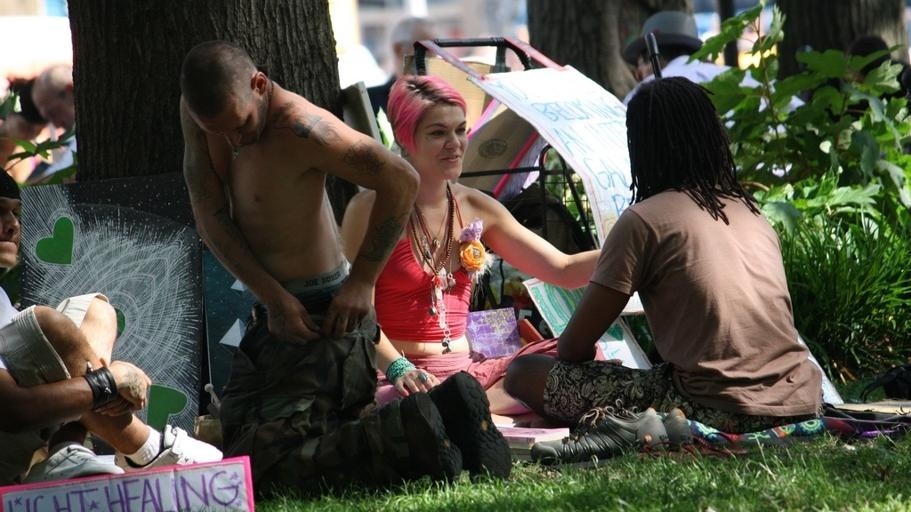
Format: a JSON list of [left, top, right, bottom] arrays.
[[419, 370, 429, 383]]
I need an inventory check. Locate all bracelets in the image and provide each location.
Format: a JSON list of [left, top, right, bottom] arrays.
[[386, 356, 415, 381], [86, 364, 121, 412]]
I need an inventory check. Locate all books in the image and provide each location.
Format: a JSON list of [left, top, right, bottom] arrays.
[[493, 423, 572, 460], [339, 82, 383, 146]]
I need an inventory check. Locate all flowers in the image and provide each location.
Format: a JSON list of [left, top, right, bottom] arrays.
[[459, 217, 496, 285]]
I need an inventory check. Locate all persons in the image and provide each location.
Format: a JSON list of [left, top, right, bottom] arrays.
[[28, 65, 80, 182], [366, 17, 444, 114], [500, 75, 826, 440], [337, 69, 613, 458], [0, 76, 54, 184], [617, 11, 811, 131], [1, 168, 224, 484], [177, 36, 515, 499]]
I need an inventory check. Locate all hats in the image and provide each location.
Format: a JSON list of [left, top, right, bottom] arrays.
[[624, 10, 703, 62]]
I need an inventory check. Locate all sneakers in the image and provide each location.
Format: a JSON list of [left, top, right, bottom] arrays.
[[25, 444, 126, 485], [432, 370, 512, 485], [114, 424, 223, 471], [400, 392, 462, 483], [530, 404, 694, 467]]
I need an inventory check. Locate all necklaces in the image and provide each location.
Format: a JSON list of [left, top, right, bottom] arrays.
[[408, 178, 464, 359]]
